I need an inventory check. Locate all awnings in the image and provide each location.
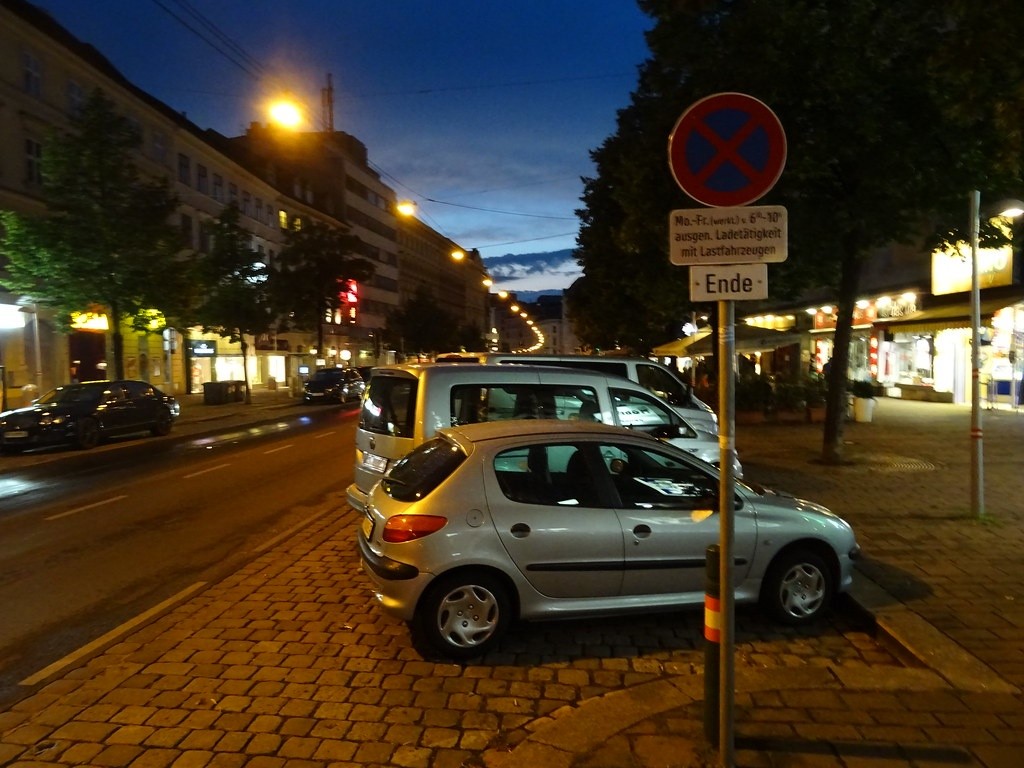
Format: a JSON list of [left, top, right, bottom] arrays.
[[872, 293, 1023, 340], [653, 314, 872, 359]]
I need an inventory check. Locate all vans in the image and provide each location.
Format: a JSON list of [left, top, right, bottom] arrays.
[[345, 358, 746, 513], [438, 351, 719, 440]]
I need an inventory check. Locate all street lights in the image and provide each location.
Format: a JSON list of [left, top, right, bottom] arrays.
[[690, 310, 709, 390]]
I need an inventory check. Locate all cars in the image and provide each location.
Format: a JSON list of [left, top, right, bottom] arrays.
[[301, 367, 367, 405], [0, 377, 183, 458], [353, 418, 861, 662]]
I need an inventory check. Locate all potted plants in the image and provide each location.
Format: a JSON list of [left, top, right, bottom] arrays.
[[850, 380, 878, 422]]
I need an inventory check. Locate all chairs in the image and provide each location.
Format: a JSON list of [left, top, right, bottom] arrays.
[[580, 400, 598, 419], [537, 392, 559, 419], [512, 392, 539, 419], [527, 448, 553, 503], [565, 451, 598, 504]]
